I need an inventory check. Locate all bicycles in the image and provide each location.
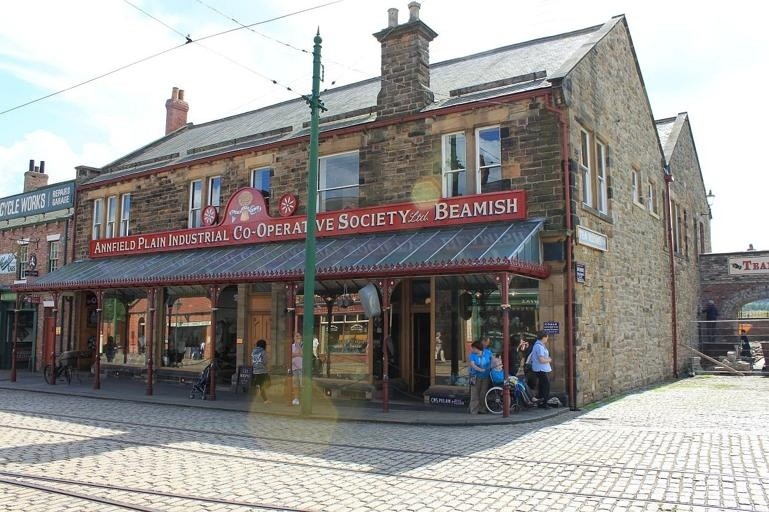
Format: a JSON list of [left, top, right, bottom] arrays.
[[42, 352, 73, 385]]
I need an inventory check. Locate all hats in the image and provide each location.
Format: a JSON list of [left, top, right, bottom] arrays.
[[527, 398, 552, 409], [491, 355, 502, 368]]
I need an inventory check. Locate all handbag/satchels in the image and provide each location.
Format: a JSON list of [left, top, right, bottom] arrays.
[[469, 376, 477, 385], [526, 353, 533, 366]]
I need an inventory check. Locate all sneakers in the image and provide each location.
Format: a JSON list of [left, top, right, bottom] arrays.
[[264, 399, 272, 404], [256, 384, 262, 395], [292, 399, 299, 406]]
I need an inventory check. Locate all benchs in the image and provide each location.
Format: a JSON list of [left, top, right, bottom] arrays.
[[91, 352, 216, 400]]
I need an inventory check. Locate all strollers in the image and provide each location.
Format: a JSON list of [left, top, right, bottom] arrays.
[[184, 358, 217, 401]]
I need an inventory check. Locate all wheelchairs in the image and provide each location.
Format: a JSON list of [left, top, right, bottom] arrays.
[[483, 364, 544, 417]]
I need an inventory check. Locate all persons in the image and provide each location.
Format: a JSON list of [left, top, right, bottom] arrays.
[[741, 330, 751, 361], [435, 332, 446, 363], [702, 300, 718, 342], [292, 330, 303, 405], [251, 340, 273, 404], [102, 336, 118, 363], [467, 330, 553, 417]]
